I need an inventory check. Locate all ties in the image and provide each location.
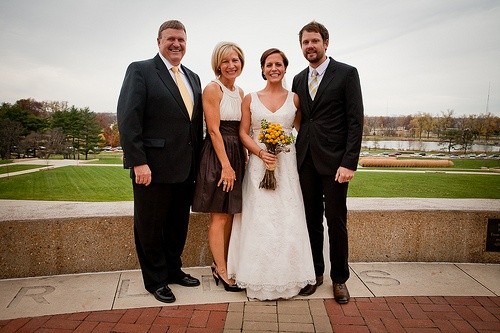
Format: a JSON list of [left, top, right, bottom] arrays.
[[170, 67, 194, 122], [308, 70, 319, 102]]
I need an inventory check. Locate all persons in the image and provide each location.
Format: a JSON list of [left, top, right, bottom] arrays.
[[190, 41, 249, 292], [291, 22, 365, 305], [226, 47, 332, 301], [116, 20, 203, 304]]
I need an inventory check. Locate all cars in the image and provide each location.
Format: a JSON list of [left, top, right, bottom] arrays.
[[436, 152, 498, 159], [382, 151, 402, 157], [101, 145, 123, 153]]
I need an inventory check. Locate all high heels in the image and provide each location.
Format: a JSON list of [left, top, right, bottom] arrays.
[[211, 262, 242, 291]]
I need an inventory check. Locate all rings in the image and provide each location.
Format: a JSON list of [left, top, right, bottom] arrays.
[[347, 179, 350, 181], [223, 181, 227, 185]]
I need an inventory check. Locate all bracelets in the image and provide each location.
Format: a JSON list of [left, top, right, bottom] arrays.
[[258, 149, 263, 158]]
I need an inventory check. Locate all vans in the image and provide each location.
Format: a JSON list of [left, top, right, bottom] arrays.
[[419, 152, 426, 156]]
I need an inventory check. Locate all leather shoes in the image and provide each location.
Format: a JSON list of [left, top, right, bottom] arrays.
[[168, 271, 201, 286], [154, 287, 176, 303], [333, 280, 350, 303], [299, 275, 324, 295]]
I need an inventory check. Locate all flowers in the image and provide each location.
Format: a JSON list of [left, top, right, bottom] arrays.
[[257, 119, 295, 191]]
